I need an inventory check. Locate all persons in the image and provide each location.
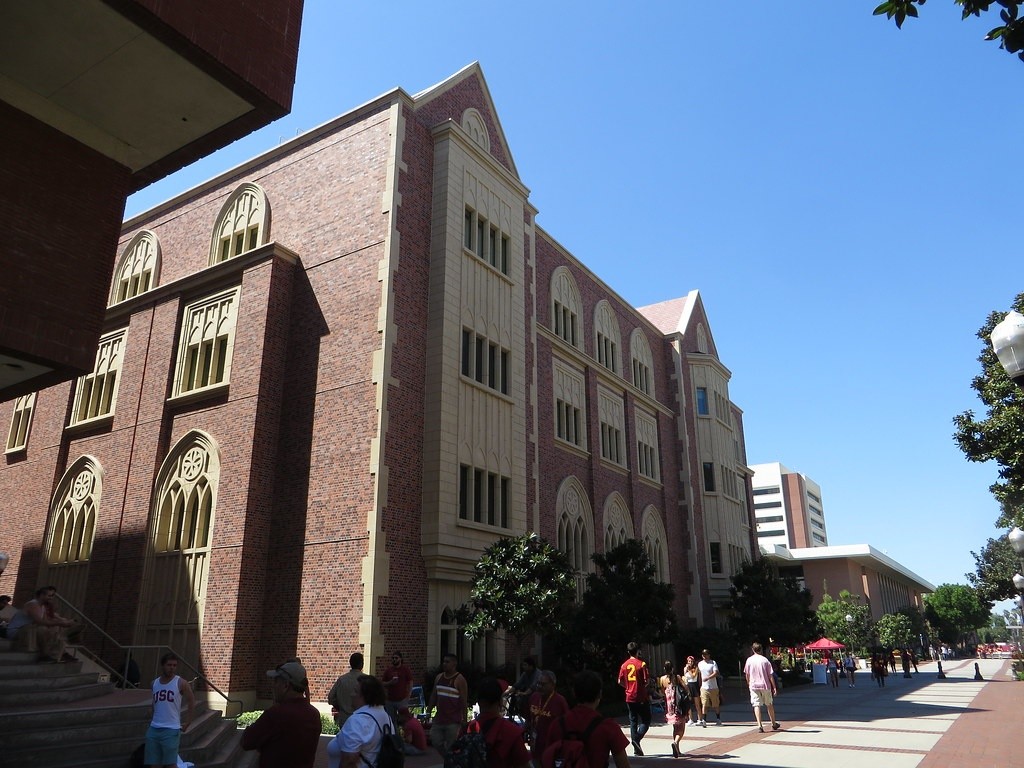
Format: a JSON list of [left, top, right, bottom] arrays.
[[457, 679, 531, 768], [683, 649, 721, 728], [327, 653, 367, 728], [503, 659, 542, 696], [0, 586, 80, 662], [929, 644, 952, 662], [618, 642, 651, 756], [524, 671, 570, 768], [540, 671, 631, 768], [326, 675, 395, 768], [844, 652, 856, 688], [977, 645, 1003, 660], [240, 656, 322, 768], [737, 643, 780, 732], [787, 652, 792, 668], [426, 653, 468, 760], [381, 651, 427, 754], [872, 649, 919, 687], [826, 654, 839, 687], [143, 653, 196, 768], [659, 660, 691, 757], [776, 652, 782, 659]]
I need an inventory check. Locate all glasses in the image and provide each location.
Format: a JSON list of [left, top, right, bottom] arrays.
[[537, 681, 550, 687], [392, 657, 401, 660], [276, 663, 300, 679]]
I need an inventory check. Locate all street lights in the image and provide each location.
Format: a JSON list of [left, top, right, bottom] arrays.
[[846, 614, 856, 659], [1013, 573, 1024, 622], [1008, 526, 1024, 571]]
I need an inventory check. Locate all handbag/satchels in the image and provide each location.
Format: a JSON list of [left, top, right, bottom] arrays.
[[675, 677, 691, 717]]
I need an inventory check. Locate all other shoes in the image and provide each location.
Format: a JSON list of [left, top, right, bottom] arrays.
[[700, 720, 707, 728], [696, 720, 701, 726], [759, 727, 764, 732], [631, 740, 643, 755], [672, 743, 679, 758], [716, 719, 721, 726], [772, 723, 780, 730], [60, 654, 78, 662], [39, 656, 57, 663]]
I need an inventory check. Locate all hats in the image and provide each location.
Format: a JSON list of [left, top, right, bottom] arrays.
[[701, 649, 710, 654], [267, 662, 306, 688]]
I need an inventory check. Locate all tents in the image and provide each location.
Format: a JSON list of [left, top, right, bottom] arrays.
[[803, 637, 845, 660]]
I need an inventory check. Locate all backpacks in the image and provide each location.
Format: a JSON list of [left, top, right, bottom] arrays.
[[443, 717, 498, 768], [354, 712, 405, 768], [542, 713, 605, 768]]
